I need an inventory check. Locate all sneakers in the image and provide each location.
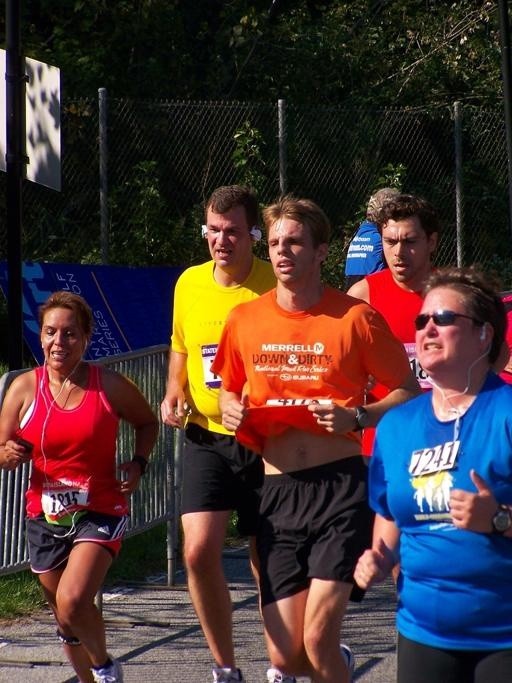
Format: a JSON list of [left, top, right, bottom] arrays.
[[89, 653, 123, 683], [213, 666, 246, 683]]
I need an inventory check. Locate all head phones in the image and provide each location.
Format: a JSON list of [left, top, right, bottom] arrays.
[[201, 224, 208, 241]]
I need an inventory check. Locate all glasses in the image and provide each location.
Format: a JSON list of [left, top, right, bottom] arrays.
[[415, 309, 478, 329]]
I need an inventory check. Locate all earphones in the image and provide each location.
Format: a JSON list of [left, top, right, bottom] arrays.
[[480, 323, 487, 341], [250, 225, 262, 242], [85, 339, 88, 351]]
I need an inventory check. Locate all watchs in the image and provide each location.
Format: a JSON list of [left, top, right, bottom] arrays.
[[492, 505, 511, 537], [353, 406, 370, 432], [132, 454, 149, 477]]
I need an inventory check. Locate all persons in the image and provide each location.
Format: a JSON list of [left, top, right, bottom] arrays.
[[161, 182, 296, 683], [347, 194, 444, 592], [209, 197, 423, 683], [355, 264, 511, 683], [1, 292, 159, 683], [347, 188, 403, 287]]
